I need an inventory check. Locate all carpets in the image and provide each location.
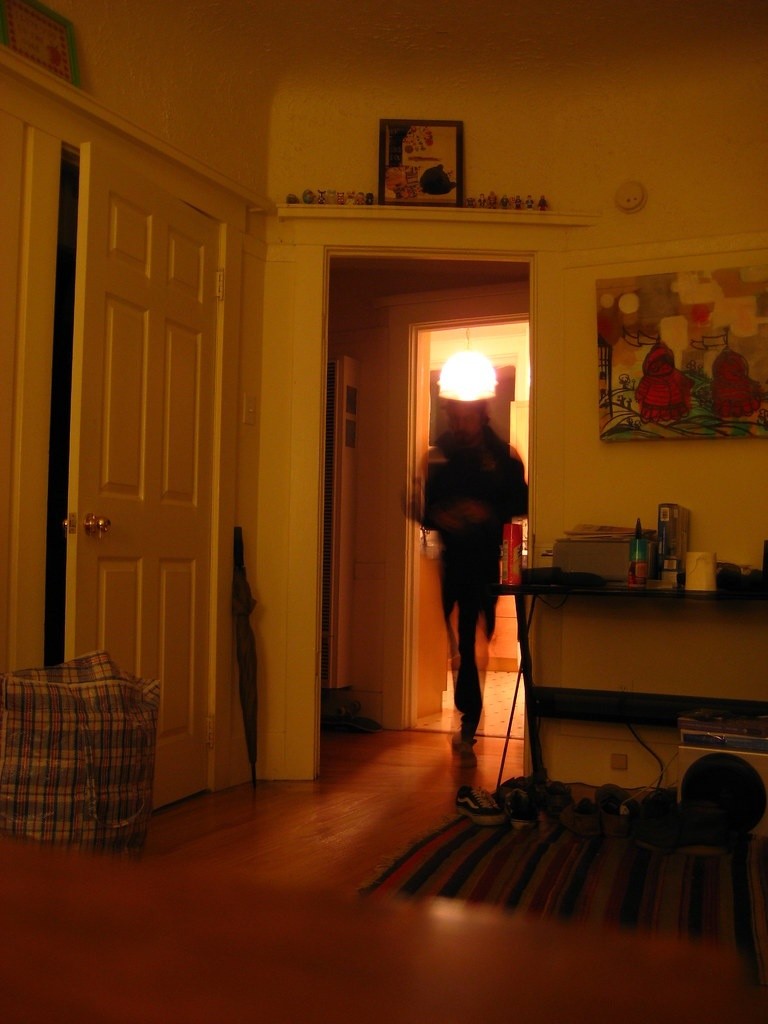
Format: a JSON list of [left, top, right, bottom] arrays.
[[359, 773, 768, 986]]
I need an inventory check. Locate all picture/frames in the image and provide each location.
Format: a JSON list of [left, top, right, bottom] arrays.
[[0, 0, 82, 89], [378, 119, 464, 207]]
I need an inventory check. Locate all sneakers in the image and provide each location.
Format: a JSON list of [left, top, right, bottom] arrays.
[[501, 779, 537, 830], [454, 785, 506, 826]]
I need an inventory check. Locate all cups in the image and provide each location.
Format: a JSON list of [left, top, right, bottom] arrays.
[[685, 551, 717, 591]]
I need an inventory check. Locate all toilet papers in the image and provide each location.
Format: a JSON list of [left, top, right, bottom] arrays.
[[685, 551, 717, 592]]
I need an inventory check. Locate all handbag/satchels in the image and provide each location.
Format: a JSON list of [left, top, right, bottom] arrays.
[[0, 650, 159, 848]]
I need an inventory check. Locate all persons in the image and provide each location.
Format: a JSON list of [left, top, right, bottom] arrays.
[[402, 398, 529, 756]]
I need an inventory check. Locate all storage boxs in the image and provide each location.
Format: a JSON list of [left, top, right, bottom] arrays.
[[658, 503, 691, 572], [553, 541, 659, 581]]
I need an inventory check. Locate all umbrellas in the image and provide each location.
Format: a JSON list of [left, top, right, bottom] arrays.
[[230, 525, 257, 790]]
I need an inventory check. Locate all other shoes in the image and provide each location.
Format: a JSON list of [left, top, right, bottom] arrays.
[[452, 736, 477, 768], [545, 780, 572, 815], [559, 797, 601, 837], [596, 785, 632, 840], [636, 786, 679, 851]]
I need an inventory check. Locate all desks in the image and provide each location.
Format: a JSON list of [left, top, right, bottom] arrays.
[[0, 832, 760, 1022], [496, 582, 768, 784]]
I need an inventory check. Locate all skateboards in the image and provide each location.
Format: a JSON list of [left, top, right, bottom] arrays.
[[319, 700, 381, 733]]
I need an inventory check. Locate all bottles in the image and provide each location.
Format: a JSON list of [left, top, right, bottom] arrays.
[[501, 524, 523, 585], [627, 519, 648, 560], [655, 523, 666, 580]]
